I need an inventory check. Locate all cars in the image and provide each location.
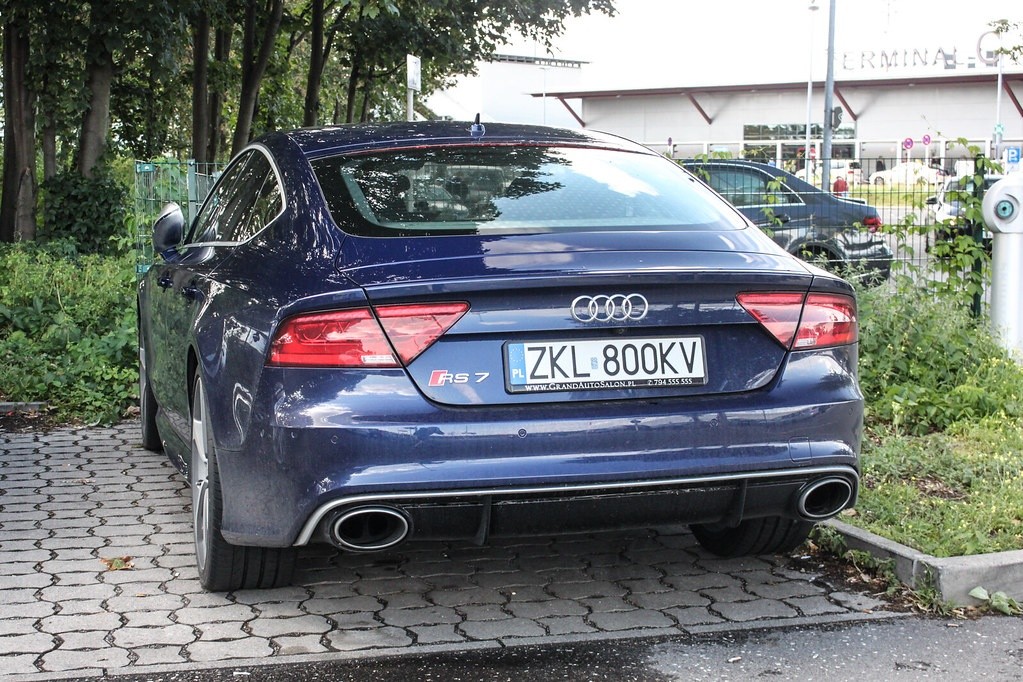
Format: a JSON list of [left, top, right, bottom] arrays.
[[869, 160, 947, 191], [676, 155, 893, 290], [132, 114, 867, 591], [795, 157, 864, 188]]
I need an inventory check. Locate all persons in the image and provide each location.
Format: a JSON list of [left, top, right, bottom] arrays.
[[833, 176, 849, 197]]
